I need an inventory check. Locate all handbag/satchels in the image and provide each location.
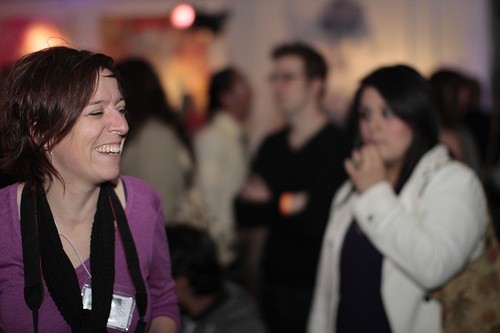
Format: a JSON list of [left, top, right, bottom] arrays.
[[426, 238, 500, 332]]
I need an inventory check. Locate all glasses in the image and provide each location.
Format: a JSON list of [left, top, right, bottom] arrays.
[[268, 72, 313, 83]]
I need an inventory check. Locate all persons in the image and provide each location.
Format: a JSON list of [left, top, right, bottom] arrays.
[[192, 65, 255, 289], [232, 40, 358, 333], [429, 67, 499, 242], [160, 222, 271, 333], [111, 55, 211, 232], [0, 44, 182, 333], [301, 64, 499, 333]]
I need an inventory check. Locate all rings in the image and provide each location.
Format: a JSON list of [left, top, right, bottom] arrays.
[[354, 161, 361, 169]]
